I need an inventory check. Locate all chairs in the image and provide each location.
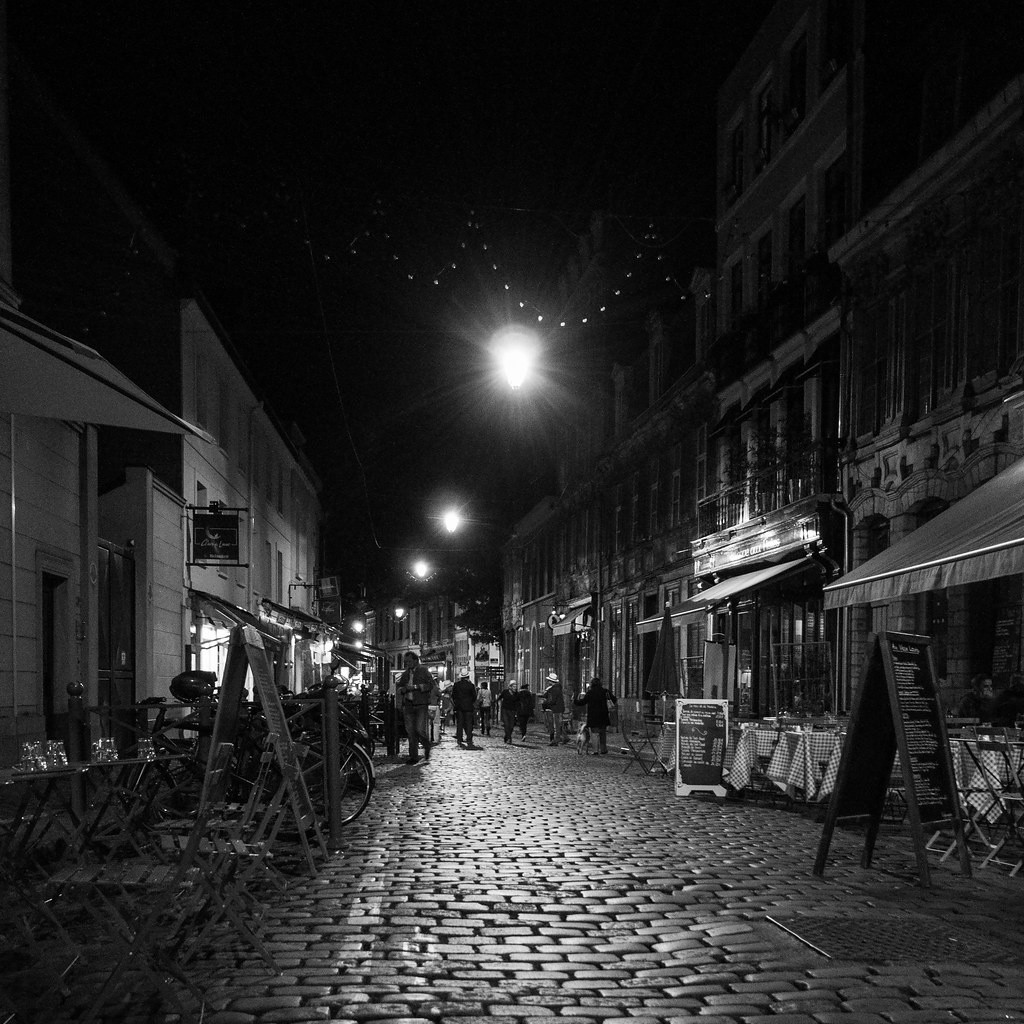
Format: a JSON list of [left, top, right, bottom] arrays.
[[620, 720, 649, 776], [923, 718, 1003, 863], [643, 711, 672, 779], [974, 726, 1024, 876], [1, 731, 330, 1024], [754, 716, 812, 808]]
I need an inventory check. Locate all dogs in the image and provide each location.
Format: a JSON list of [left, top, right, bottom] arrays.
[[577, 722, 590, 756]]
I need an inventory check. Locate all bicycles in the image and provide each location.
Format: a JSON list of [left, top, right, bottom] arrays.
[[83, 658, 374, 857]]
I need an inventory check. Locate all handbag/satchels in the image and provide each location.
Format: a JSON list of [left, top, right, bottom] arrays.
[[607, 690, 615, 709], [477, 694, 483, 708]]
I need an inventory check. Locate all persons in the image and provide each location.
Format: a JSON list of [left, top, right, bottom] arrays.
[[518, 684, 535, 741], [398, 652, 434, 764], [574, 678, 617, 754], [477, 682, 493, 736], [451, 669, 477, 749], [959, 673, 1024, 727], [430, 676, 457, 725], [537, 673, 570, 747], [493, 680, 519, 744], [360, 684, 370, 697]]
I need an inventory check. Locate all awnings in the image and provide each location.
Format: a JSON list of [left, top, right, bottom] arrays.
[[199, 592, 280, 645], [636, 557, 809, 635], [264, 599, 322, 624], [822, 457, 1024, 609], [0, 302, 215, 444], [552, 605, 591, 636]]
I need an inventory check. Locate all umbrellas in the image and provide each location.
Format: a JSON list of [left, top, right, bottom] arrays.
[[645, 602, 679, 721]]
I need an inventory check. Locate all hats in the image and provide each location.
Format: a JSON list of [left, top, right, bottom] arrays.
[[546, 673, 560, 683], [459, 668, 470, 677], [509, 679, 517, 686]]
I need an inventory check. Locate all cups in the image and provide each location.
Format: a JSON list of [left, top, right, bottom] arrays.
[[803, 723, 812, 732], [45, 740, 68, 767], [20, 741, 48, 771], [137, 737, 157, 759], [92, 737, 119, 762]]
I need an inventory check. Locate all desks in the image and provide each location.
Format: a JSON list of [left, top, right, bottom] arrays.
[[647, 716, 1024, 878], [0, 761, 101, 879], [81, 755, 189, 862]]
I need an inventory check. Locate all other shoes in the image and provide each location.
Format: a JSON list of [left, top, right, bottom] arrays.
[[601, 750, 608, 754], [591, 752, 599, 756], [562, 739, 570, 745], [521, 735, 527, 742], [405, 759, 418, 765], [546, 741, 559, 746]]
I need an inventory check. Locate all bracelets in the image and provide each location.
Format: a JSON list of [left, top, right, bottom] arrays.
[[415, 685, 417, 689]]
